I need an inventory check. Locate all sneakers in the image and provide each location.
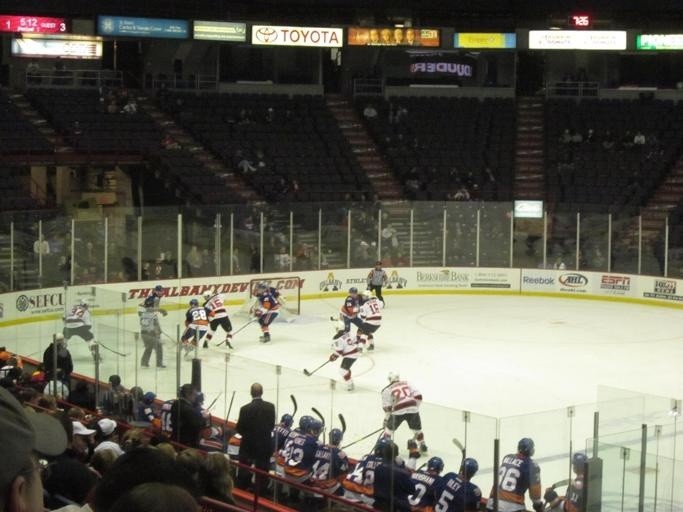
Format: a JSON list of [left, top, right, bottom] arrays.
[[366, 345, 374, 350], [139, 333, 271, 369]]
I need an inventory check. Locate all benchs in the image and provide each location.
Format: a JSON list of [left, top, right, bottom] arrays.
[[0, 90, 682, 275], [0, 383, 378, 512]]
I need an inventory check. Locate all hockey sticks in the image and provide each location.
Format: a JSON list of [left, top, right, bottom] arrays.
[[303, 359, 330, 376], [453, 438, 465, 474], [330, 316, 341, 322], [291, 395, 346, 446], [208, 319, 254, 347], [542, 479, 573, 510], [97, 339, 133, 356]]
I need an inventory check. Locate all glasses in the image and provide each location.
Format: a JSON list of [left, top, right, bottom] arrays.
[[24, 459, 58, 483]]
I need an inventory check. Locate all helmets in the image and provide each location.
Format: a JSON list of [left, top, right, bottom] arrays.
[[81, 298, 90, 308], [280, 414, 343, 443], [189, 290, 212, 306], [517, 437, 535, 456], [335, 285, 371, 332], [571, 452, 589, 473], [387, 370, 399, 384], [460, 458, 479, 480], [144, 285, 164, 307], [428, 456, 444, 472]]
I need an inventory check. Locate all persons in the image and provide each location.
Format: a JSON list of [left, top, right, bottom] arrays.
[[1, 347, 233, 512], [329, 320, 359, 391], [539, 247, 606, 270], [160, 132, 180, 150], [364, 103, 408, 124], [140, 299, 166, 368], [437, 198, 512, 266], [467, 171, 478, 189], [407, 173, 420, 188], [137, 285, 167, 349], [340, 287, 368, 335], [181, 299, 215, 352], [34, 213, 326, 285], [96, 85, 139, 114], [559, 128, 595, 143], [68, 120, 83, 143], [52, 57, 66, 84], [251, 282, 280, 301], [28, 58, 40, 85], [366, 261, 389, 309], [236, 107, 293, 125], [158, 83, 168, 97], [353, 289, 384, 351], [203, 290, 234, 350], [276, 178, 299, 200], [621, 128, 656, 148], [234, 148, 271, 172], [236, 375, 588, 511], [62, 298, 103, 363], [250, 285, 281, 343], [43, 333, 74, 381], [337, 191, 401, 267]]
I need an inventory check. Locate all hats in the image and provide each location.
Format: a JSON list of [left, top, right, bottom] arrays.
[[0, 386, 117, 495]]
